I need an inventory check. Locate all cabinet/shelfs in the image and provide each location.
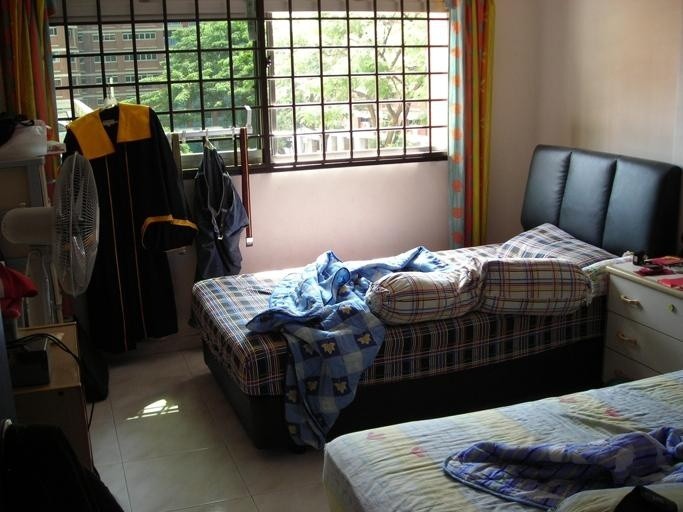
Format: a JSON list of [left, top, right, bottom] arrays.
[[0, 154, 62, 330], [7, 321, 95, 475]]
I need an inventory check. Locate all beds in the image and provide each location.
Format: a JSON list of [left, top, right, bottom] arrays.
[[322, 366, 683, 511], [189, 145, 683, 456]]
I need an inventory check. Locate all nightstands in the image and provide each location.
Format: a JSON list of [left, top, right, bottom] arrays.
[[601, 264, 683, 384]]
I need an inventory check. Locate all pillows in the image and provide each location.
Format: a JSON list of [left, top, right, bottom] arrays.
[[472, 222, 648, 317], [365, 265, 479, 325], [557, 484, 683, 512]]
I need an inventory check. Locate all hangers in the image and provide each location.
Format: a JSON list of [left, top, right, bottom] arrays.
[[97, 76, 120, 127]]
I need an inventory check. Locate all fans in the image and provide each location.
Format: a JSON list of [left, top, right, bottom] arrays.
[[2, 152, 101, 327]]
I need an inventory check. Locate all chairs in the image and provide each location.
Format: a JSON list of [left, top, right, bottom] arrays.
[[0, 420, 122, 511]]
